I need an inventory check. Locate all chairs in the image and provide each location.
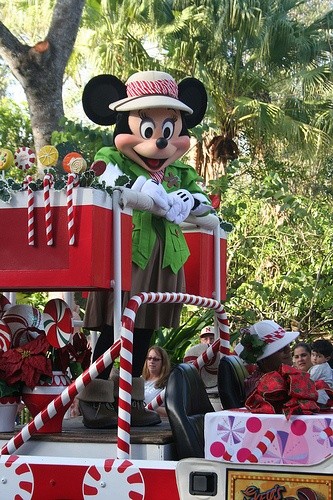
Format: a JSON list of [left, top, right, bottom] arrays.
[[218, 355, 249, 410], [165, 363, 215, 459]]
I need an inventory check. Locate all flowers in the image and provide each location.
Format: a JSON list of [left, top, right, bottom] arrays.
[[0, 332, 91, 391]]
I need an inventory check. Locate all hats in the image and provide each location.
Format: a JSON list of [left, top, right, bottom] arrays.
[[245, 320, 300, 363], [199, 325, 214, 337]]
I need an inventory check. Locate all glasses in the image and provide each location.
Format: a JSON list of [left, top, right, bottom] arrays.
[[147, 356, 163, 362]]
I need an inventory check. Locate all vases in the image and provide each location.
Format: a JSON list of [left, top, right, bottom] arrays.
[[0, 394, 21, 432], [23, 371, 73, 432]]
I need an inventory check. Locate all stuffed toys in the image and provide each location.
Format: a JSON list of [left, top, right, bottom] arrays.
[[77, 71, 211, 428]]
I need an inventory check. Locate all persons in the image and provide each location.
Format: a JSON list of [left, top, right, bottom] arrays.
[[293, 343, 313, 373], [307, 340, 333, 405], [240, 319, 300, 400], [200, 326, 214, 347], [140, 346, 170, 417]]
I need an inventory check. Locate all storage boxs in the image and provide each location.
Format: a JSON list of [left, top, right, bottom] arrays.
[[204, 406, 333, 467]]
